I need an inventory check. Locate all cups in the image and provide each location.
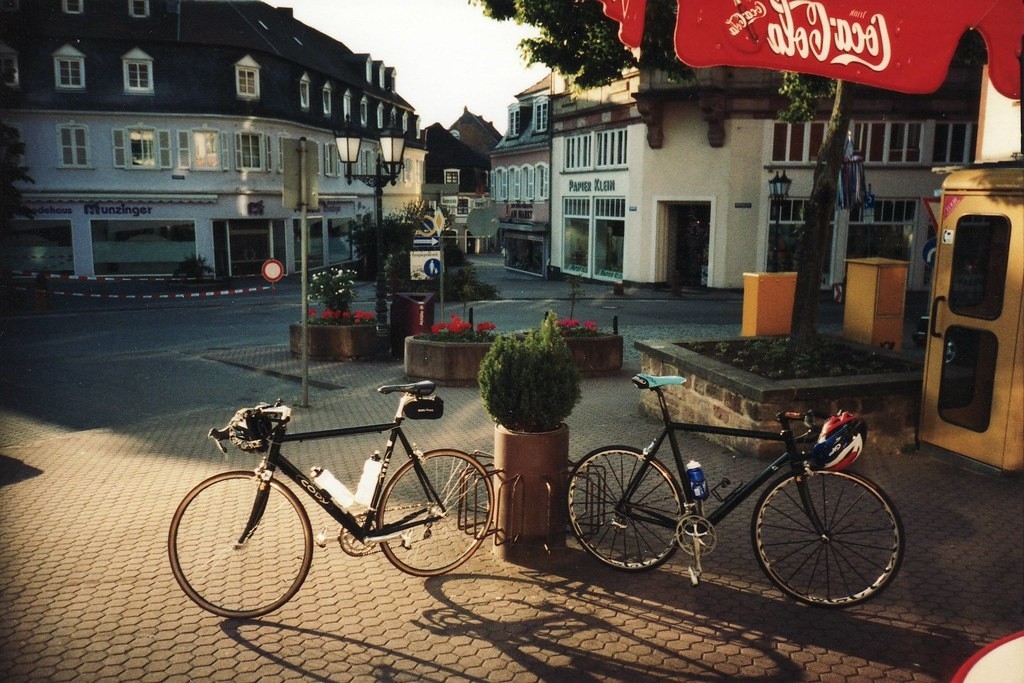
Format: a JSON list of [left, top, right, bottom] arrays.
[[687, 468, 707, 500]]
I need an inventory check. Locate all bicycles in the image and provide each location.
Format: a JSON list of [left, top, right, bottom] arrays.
[[566, 372, 907, 610], [168, 379, 494, 620]]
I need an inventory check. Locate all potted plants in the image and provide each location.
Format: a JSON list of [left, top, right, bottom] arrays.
[[476, 307, 585, 563]]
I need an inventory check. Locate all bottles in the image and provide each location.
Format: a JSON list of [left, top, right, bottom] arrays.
[[686, 458, 702, 473], [310, 466, 355, 509], [355, 451, 382, 506]]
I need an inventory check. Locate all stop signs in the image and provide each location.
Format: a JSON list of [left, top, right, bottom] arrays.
[[261, 259, 284, 283]]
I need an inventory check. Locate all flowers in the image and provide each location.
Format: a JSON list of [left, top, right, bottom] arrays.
[[418, 313, 506, 343], [305, 308, 378, 327], [307, 268, 360, 308], [542, 316, 612, 338]]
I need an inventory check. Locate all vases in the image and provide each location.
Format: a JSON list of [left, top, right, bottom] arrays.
[[403, 336, 511, 386], [288, 322, 393, 361], [328, 307, 353, 314], [517, 329, 624, 377]]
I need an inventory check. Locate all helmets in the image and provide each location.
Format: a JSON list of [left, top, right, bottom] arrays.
[[228, 408, 272, 451], [808, 411, 867, 471]]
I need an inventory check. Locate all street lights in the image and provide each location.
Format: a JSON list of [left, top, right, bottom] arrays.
[[330, 111, 407, 356], [768, 169, 792, 272]]
